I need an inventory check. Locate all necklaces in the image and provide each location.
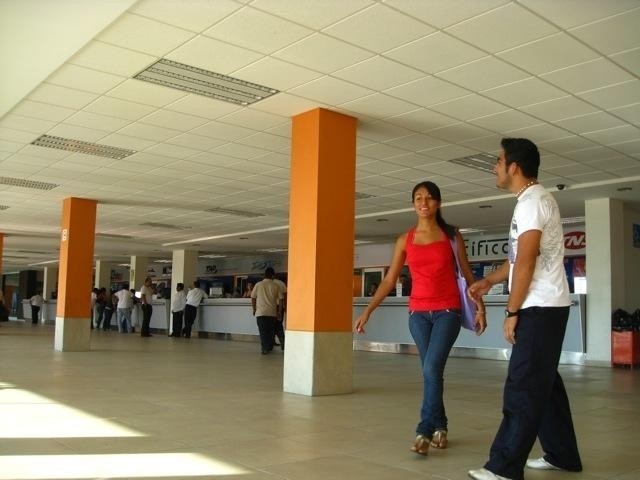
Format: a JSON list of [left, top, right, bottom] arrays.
[[513, 180, 539, 197]]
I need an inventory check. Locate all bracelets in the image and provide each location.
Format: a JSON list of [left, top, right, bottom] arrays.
[[475, 310, 485, 314]]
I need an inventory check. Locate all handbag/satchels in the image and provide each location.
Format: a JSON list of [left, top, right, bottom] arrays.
[[459, 276, 479, 332]]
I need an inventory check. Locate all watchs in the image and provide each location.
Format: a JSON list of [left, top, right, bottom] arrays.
[[504, 310, 518, 317]]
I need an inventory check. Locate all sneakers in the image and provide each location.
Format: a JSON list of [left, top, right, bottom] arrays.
[[526, 456, 562, 470], [468, 466, 510, 480]]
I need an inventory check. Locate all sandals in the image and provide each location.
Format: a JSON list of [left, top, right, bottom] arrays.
[[410, 434, 430, 456], [431, 430, 448, 449]]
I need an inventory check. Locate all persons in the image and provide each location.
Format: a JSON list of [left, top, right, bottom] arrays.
[[30, 291, 44, 323], [183, 281, 208, 337], [241, 267, 287, 355], [168, 283, 187, 337], [92, 277, 171, 336], [467, 137, 583, 480], [391, 275, 410, 296], [356, 180, 488, 454]]
